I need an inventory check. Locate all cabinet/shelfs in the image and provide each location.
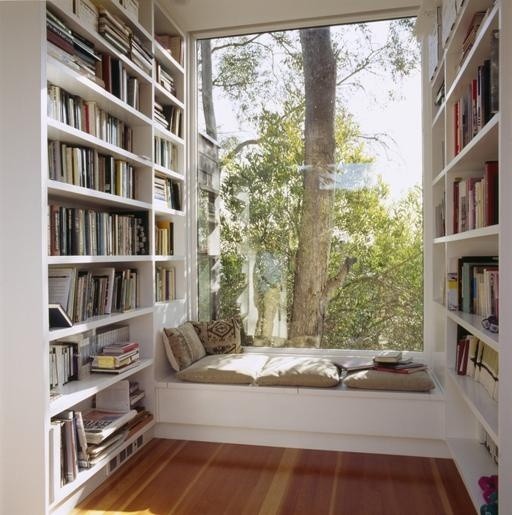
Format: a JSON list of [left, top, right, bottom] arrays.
[[430, 0, 512, 515], [0, 0, 189, 515]]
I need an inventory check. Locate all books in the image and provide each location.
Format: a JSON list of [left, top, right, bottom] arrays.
[[331, 351, 428, 374], [428, 11, 499, 464], [154, 171, 181, 212], [48, 323, 142, 398], [46, 0, 177, 95], [47, 379, 155, 504], [48, 204, 149, 257], [47, 139, 138, 200], [156, 264, 176, 301], [155, 99, 180, 175], [46, 80, 139, 155], [48, 264, 140, 331]]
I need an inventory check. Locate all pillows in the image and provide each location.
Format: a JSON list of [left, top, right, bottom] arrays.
[[161, 315, 436, 389]]
